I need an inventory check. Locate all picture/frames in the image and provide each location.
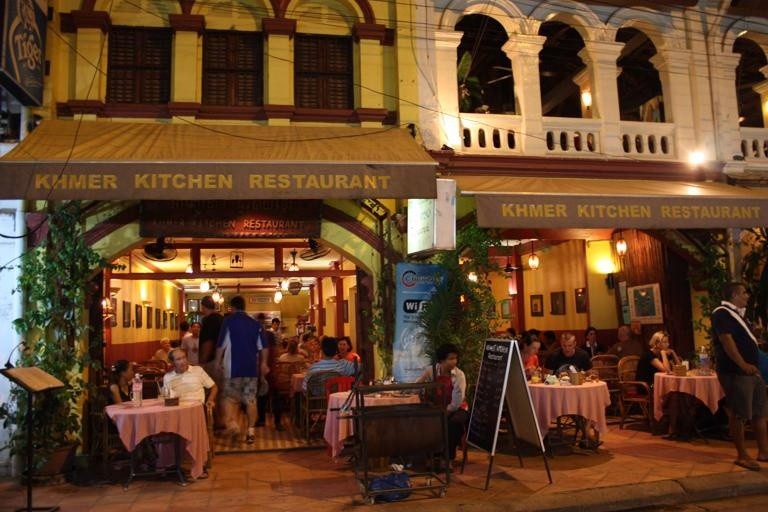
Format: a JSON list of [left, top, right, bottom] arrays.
[[529, 286, 588, 318], [108, 299, 179, 332]]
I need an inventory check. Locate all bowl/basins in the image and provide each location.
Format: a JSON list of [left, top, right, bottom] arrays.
[[164, 397, 180, 406]]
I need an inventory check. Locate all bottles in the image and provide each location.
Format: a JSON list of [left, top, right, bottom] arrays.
[[698, 345, 712, 376], [131, 373, 143, 408]]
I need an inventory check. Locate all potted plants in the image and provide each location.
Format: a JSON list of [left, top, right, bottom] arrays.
[[3, 215, 125, 485]]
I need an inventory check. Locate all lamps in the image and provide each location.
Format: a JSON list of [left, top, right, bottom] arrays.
[[580, 88, 593, 108], [526, 242, 541, 274], [271, 249, 302, 305], [185, 253, 228, 306], [615, 229, 627, 261]]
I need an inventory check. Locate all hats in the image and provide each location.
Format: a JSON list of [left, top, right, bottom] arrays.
[[258, 376, 269, 397]]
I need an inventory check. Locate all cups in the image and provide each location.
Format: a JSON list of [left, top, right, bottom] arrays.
[[591, 370, 599, 383], [673, 365, 687, 376], [530, 370, 542, 384], [569, 372, 583, 385]]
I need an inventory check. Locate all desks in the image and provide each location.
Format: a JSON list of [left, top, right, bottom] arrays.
[[100, 397, 210, 495], [526, 374, 611, 459], [325, 389, 419, 457], [652, 368, 726, 440]]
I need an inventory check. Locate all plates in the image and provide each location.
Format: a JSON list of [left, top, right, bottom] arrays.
[[394, 393, 410, 398]]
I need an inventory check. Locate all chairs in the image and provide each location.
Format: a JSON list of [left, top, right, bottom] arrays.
[[125, 371, 168, 406], [617, 353, 658, 433], [589, 353, 622, 419]]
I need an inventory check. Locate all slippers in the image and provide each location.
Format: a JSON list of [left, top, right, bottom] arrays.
[[734, 460, 758, 470]]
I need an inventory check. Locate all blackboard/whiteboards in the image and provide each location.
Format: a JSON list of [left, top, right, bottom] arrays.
[[464, 338, 515, 456]]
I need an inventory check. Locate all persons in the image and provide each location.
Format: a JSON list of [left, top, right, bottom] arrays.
[[757, 347, 768, 386], [413, 343, 469, 474], [711, 281, 768, 470], [154, 295, 362, 480], [107, 359, 168, 480], [506, 320, 693, 448]]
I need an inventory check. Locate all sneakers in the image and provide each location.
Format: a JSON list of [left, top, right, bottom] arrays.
[[245, 432, 255, 442]]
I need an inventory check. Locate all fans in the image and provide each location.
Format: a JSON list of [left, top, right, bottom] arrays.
[[138, 232, 178, 263], [299, 239, 331, 262], [472, 239, 540, 277]]
